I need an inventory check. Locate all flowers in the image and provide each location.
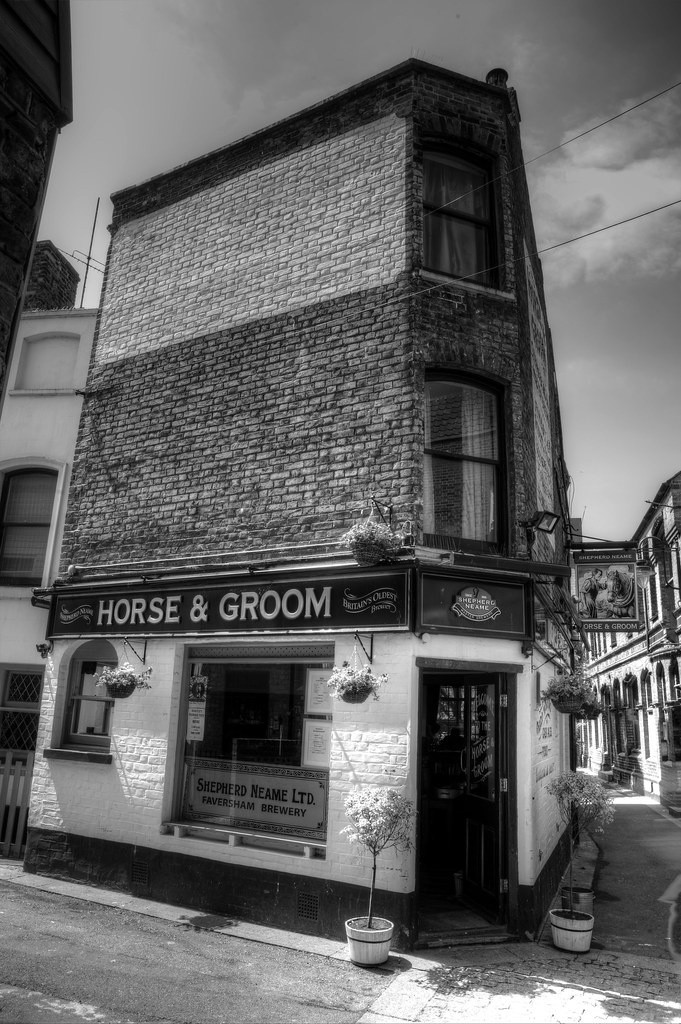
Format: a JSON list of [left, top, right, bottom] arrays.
[[339, 522, 401, 562], [539, 672, 612, 722], [326, 660, 390, 702], [91, 660, 155, 693]]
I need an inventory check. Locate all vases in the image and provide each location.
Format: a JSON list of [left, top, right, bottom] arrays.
[[576, 705, 592, 720], [105, 683, 136, 700], [551, 696, 584, 713], [588, 710, 600, 720], [342, 688, 371, 704], [351, 543, 387, 566]]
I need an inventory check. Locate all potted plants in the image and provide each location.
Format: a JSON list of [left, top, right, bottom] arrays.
[[342, 786, 418, 966], [545, 767, 615, 954]]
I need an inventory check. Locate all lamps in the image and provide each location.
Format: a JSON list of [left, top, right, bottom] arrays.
[[520, 509, 560, 556], [636, 536, 681, 603]]
